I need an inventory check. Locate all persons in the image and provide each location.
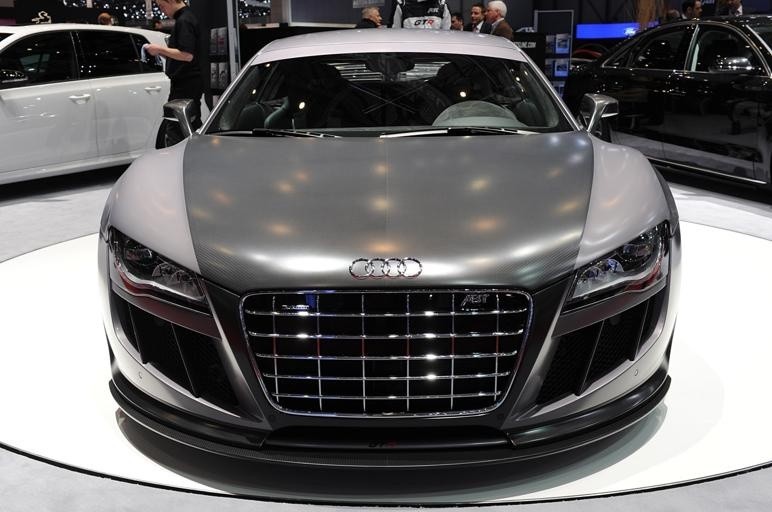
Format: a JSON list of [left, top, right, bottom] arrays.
[[143, 1, 208, 131], [264, 0, 513, 131], [666, 0, 743, 22], [97, 12, 111, 25]]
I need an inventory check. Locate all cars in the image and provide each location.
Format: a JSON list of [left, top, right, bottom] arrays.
[[95, 16, 684, 471], [563, 13, 772, 200], [0, 23, 217, 193]]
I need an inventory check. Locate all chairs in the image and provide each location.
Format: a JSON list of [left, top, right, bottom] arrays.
[[420, 61, 496, 126], [264, 62, 365, 131]]
